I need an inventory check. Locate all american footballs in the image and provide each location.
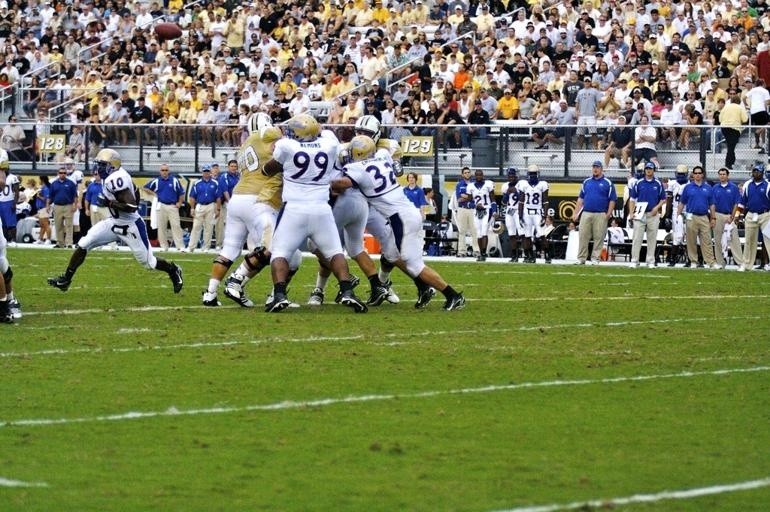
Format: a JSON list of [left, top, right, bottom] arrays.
[[154, 24, 182, 39]]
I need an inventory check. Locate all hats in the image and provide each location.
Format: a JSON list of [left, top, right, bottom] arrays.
[[645, 162, 655, 168], [202, 164, 211, 171], [753, 166, 763, 171], [592, 161, 602, 166]]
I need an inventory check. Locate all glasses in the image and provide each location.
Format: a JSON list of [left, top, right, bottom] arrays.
[[694, 172, 703, 175]]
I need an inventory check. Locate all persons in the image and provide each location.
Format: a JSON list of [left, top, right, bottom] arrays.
[[0, 216, 22, 324], [0, 0, 770, 168], [203, 113, 467, 312], [46, 148, 184, 293], [0, 162, 769, 272]]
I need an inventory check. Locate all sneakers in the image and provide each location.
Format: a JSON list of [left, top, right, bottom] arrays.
[[574, 260, 583, 265], [169, 260, 183, 293], [592, 261, 599, 265], [47, 276, 72, 291], [1, 297, 23, 321], [203, 273, 465, 312], [477, 254, 552, 264], [630, 261, 770, 273]]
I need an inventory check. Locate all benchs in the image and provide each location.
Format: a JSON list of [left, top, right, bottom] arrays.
[[616, 157, 645, 172], [144, 151, 176, 167], [724, 149, 761, 171], [433, 154, 467, 167], [523, 155, 558, 171], [222, 152, 238, 163]]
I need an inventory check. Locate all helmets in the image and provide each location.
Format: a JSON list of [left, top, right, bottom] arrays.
[[491, 221, 504, 235], [64, 159, 76, 173], [674, 164, 689, 179], [527, 163, 538, 172], [247, 111, 381, 161], [637, 163, 644, 176], [95, 148, 122, 172]]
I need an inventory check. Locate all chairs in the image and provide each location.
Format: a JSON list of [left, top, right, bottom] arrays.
[[652, 232, 686, 264], [534, 221, 569, 260], [606, 229, 631, 262], [425, 221, 450, 255]]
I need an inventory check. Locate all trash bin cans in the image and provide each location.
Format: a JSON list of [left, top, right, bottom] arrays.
[[471, 137, 498, 167]]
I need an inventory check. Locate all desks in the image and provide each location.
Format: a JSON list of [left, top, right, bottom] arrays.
[[424, 238, 458, 256]]
